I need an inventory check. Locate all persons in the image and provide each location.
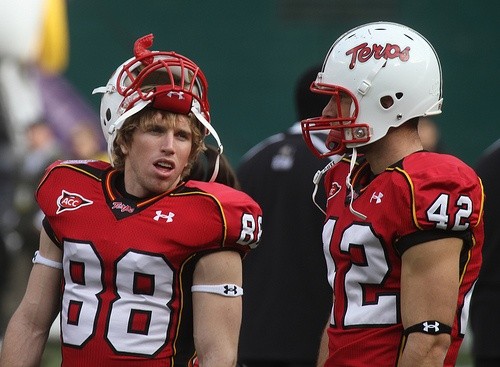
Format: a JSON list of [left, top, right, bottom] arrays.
[[236, 64, 357, 367], [0, 50, 244, 367], [415, 117, 500, 367], [317, 23, 485, 367], [177, 144, 240, 192]]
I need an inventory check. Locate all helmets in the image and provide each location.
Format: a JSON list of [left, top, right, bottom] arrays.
[[315, 21, 444, 148], [100, 50, 212, 154]]
